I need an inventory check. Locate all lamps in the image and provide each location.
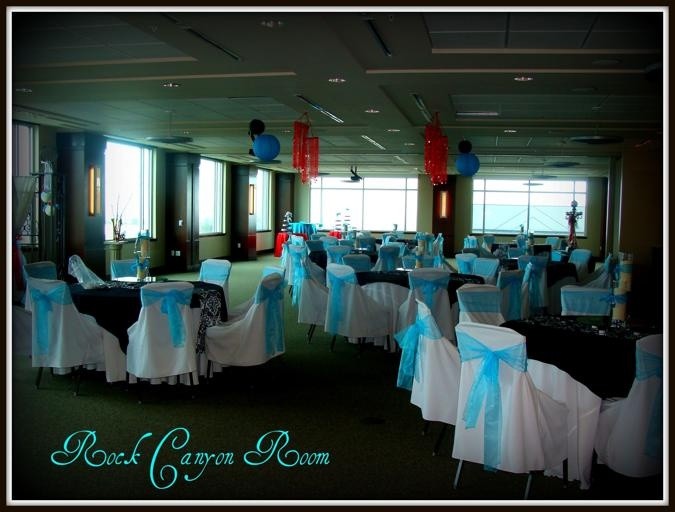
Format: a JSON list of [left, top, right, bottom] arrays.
[[248, 184, 254, 216], [88, 162, 96, 217]]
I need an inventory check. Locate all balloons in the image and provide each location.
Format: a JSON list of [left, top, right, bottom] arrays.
[[253, 134, 281, 161], [456, 153, 480, 175]]
